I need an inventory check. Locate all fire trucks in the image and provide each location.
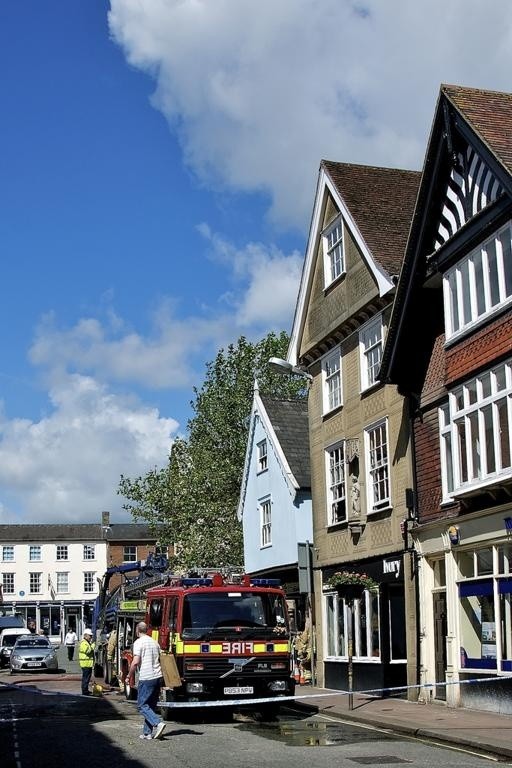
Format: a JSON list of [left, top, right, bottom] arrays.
[[90, 552, 307, 722]]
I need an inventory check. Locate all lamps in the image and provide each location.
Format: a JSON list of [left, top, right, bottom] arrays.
[[267, 353, 312, 386]]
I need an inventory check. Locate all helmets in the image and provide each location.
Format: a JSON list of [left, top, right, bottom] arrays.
[[84, 628, 94, 636]]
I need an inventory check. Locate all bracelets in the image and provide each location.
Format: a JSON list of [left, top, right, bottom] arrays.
[[127, 673, 131, 679]]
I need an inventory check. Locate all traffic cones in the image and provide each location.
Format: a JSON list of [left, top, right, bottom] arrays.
[[293, 664, 306, 684]]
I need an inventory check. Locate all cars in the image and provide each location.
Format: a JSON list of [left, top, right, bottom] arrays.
[[0, 617, 60, 674]]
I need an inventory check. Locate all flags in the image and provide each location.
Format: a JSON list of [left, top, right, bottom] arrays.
[[48, 576, 58, 601]]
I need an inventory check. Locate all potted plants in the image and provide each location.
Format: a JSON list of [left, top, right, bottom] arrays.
[[327, 570, 380, 600]]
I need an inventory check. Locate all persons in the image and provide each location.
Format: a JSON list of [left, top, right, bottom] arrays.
[[64, 629, 79, 661], [78, 628, 96, 695], [125, 621, 166, 740]]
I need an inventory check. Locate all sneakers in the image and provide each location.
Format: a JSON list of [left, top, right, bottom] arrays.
[[82, 690, 92, 696], [138, 733, 153, 741], [154, 721, 166, 739]]
